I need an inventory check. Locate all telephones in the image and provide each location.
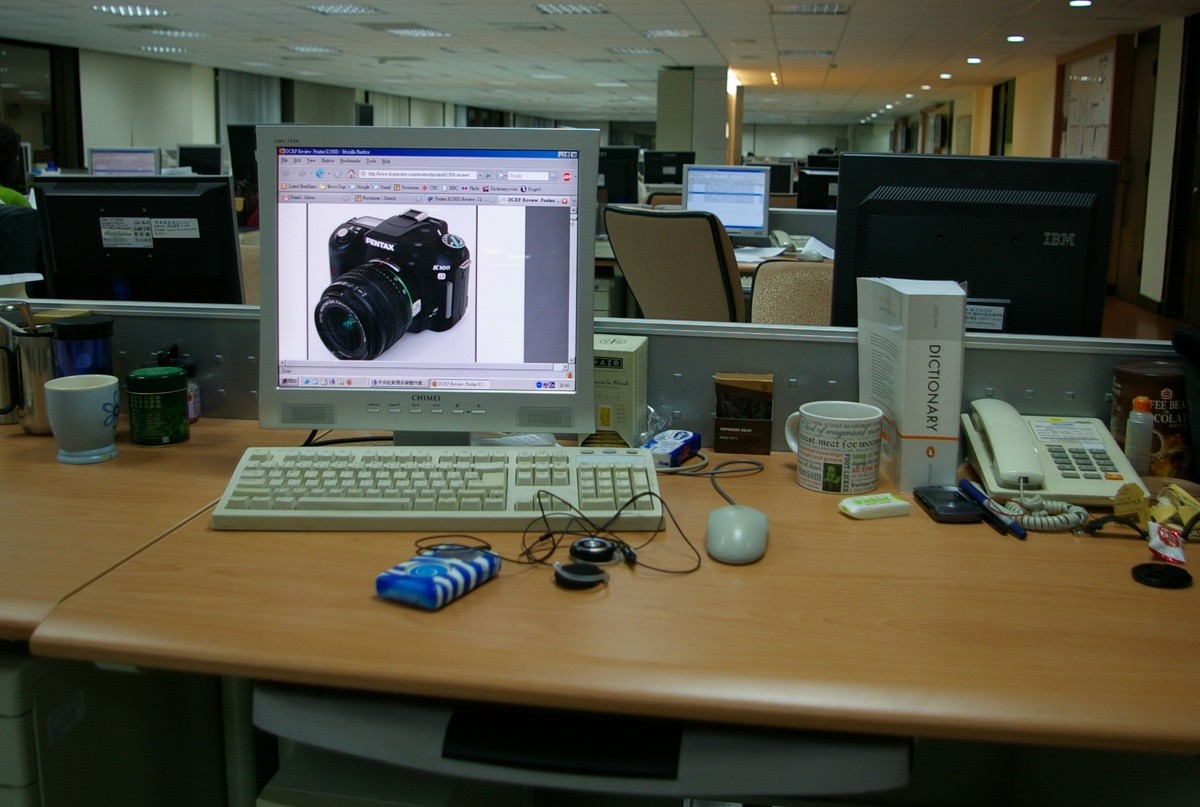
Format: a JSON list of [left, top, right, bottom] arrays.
[[960, 398, 1155, 506], [769, 229, 813, 256]]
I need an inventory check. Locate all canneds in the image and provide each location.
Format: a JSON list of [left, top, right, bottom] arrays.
[[156, 357, 201, 423]]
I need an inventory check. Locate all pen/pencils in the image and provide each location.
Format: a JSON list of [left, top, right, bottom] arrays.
[[958, 489, 1008, 535], [958, 478, 1028, 539]]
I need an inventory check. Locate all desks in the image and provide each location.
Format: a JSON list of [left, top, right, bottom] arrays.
[[0, 406, 1200, 807]]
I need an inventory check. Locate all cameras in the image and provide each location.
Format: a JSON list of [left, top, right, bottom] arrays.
[[311, 206, 470, 360]]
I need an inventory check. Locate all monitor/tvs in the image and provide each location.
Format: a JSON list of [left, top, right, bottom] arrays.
[[31, 173, 245, 307], [255, 123, 597, 445], [597, 145, 639, 232], [832, 149, 1119, 339], [643, 149, 695, 185], [87, 144, 222, 177], [742, 153, 838, 212], [682, 162, 771, 238]]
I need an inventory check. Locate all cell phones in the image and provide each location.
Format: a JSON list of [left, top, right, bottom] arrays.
[[914, 487, 980, 525]]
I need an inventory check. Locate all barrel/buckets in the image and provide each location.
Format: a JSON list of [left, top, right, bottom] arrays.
[[1108, 358, 1183, 476]]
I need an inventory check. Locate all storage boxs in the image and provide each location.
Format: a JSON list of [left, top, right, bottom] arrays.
[[578, 334, 648, 448], [639, 430, 701, 468]]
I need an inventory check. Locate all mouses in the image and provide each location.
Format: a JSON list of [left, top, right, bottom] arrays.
[[706, 505, 769, 567]]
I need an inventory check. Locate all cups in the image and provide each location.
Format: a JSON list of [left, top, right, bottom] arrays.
[[44, 374, 121, 465], [11, 323, 53, 436], [784, 400, 883, 495], [50, 316, 115, 380], [0, 301, 37, 425]]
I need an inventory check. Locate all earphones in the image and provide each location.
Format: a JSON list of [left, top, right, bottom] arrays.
[[552, 538, 622, 593]]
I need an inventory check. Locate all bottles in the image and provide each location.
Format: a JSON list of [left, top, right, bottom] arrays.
[[1123, 395, 1153, 477], [181, 361, 201, 424], [125, 367, 191, 448]]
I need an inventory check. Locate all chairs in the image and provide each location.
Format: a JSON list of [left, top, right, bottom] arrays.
[[603, 205, 834, 326]]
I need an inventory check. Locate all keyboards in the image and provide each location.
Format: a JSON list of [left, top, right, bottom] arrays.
[[212, 446, 665, 533]]
